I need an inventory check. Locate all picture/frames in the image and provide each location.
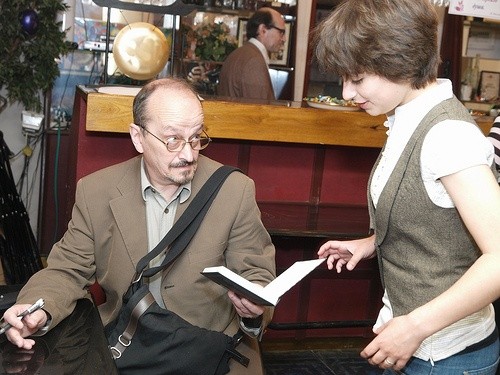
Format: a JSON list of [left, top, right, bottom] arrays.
[[236, 17, 294, 67]]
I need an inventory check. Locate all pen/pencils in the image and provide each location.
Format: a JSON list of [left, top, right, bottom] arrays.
[[0, 298, 45, 334]]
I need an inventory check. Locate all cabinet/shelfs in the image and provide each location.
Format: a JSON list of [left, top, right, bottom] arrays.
[[84, 0, 297, 81]]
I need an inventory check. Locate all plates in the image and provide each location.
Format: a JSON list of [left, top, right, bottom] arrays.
[[303, 96, 360, 112]]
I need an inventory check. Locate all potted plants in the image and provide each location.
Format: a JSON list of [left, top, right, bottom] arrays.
[[0, 0, 79, 132]]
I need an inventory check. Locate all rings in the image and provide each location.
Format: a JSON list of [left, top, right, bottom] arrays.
[[384, 359, 394, 367]]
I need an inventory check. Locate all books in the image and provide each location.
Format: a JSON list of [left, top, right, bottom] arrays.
[[200, 258, 329, 306]]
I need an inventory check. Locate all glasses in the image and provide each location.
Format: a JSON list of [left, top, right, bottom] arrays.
[[135, 123, 213, 152], [261, 23, 285, 36]]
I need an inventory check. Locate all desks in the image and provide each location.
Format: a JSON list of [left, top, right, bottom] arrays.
[[258, 201, 372, 331], [0, 284, 118, 375]]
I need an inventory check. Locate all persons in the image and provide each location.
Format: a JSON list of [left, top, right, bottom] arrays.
[[0, 79, 280, 375], [312, 0, 500, 375], [213, 7, 286, 103], [188, 62, 221, 83]]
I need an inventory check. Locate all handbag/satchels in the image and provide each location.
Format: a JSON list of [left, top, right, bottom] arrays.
[[105, 282, 251, 375]]
